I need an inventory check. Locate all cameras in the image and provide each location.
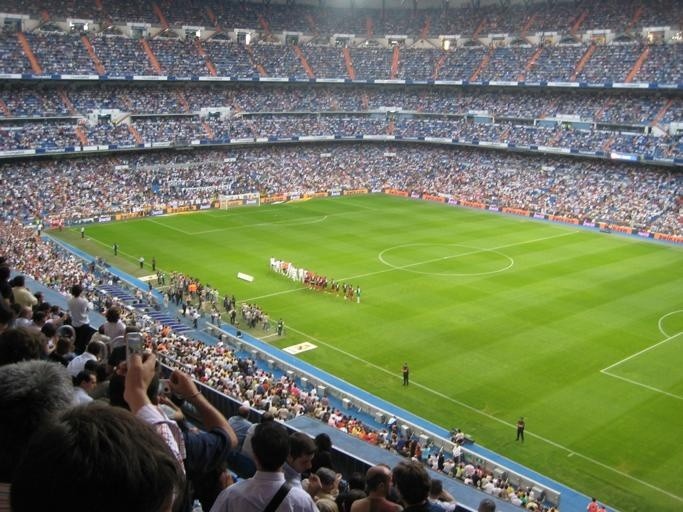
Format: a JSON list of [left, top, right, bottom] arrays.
[[156, 379, 176, 394]]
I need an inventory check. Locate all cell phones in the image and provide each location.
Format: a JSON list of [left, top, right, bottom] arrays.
[[126, 332, 144, 368]]
